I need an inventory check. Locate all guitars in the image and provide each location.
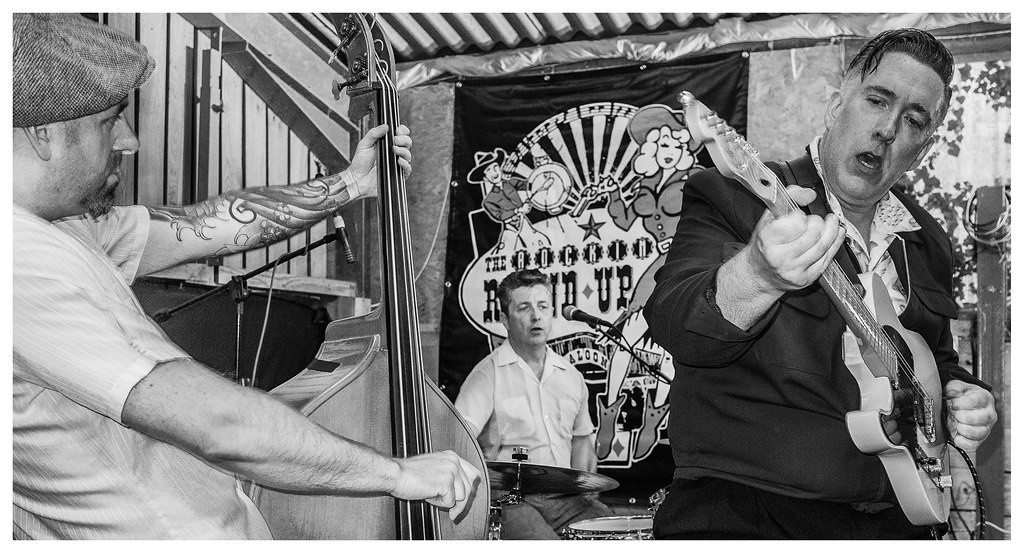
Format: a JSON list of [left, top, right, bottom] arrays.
[[677, 88, 955, 527]]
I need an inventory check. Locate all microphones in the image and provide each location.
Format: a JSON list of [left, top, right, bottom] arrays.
[[333, 210, 355, 264], [563, 305, 613, 329]]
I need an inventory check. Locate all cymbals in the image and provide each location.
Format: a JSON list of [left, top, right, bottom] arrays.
[[484, 460, 620, 497]]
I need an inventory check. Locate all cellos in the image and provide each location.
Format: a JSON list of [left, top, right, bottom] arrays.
[[234, 12, 494, 542]]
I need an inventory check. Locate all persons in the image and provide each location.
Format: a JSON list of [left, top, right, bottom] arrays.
[[642, 29, 998, 540], [452, 271, 613, 540], [13, 13, 482, 540]]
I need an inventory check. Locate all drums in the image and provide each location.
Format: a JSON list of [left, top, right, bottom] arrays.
[[568, 515, 655, 540], [650, 484, 672, 515]]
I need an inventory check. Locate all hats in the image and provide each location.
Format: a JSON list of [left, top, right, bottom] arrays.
[[13, 13, 156, 128]]
